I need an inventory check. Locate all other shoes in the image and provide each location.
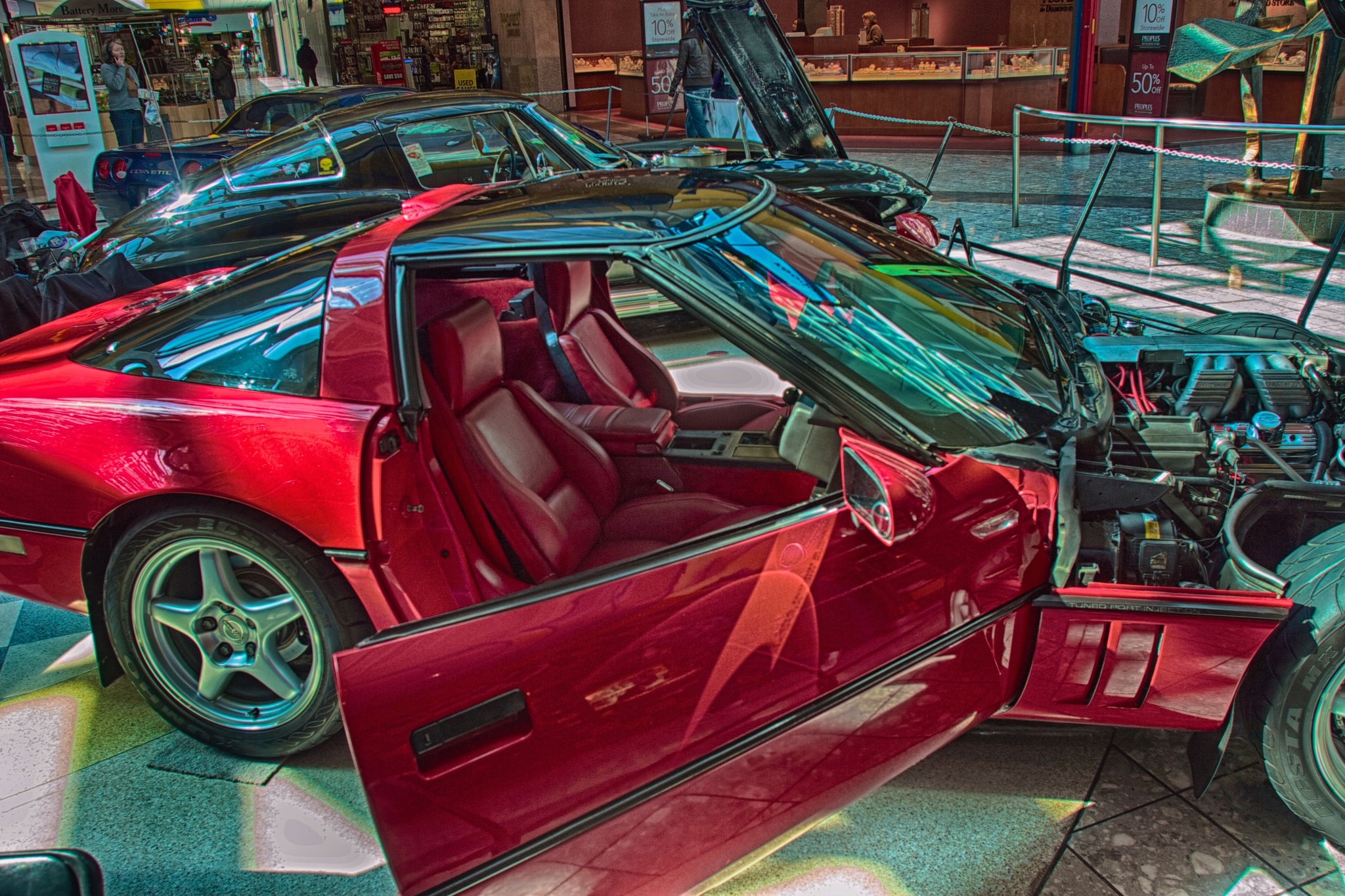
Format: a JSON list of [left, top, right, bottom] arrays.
[[6, 154, 23, 161], [246, 75, 249, 79]]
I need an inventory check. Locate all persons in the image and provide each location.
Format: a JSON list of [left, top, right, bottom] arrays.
[[135, 35, 210, 103], [856, 12, 887, 47], [296, 38, 322, 87], [451, 61, 458, 79], [668, 15, 716, 138], [412, 34, 436, 76], [240, 44, 253, 79], [101, 37, 148, 147], [791, 19, 809, 37], [484, 57, 496, 89], [209, 43, 237, 118]]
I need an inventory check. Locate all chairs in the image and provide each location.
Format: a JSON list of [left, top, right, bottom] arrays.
[[529, 259, 793, 431], [418, 292, 794, 584], [269, 112, 297, 134]]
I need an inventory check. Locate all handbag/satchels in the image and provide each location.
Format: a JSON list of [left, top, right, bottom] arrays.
[[144, 100, 161, 127]]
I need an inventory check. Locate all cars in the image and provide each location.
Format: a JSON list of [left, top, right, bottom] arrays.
[[91, 84, 602, 229]]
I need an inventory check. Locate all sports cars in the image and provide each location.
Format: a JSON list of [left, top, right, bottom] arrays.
[[3, 166, 1345, 896], [1, 0, 942, 344]]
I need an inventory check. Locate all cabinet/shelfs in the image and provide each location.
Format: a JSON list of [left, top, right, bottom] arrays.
[[163, 0, 501, 93]]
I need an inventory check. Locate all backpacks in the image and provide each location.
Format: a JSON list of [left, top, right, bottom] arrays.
[[244, 50, 252, 65]]
